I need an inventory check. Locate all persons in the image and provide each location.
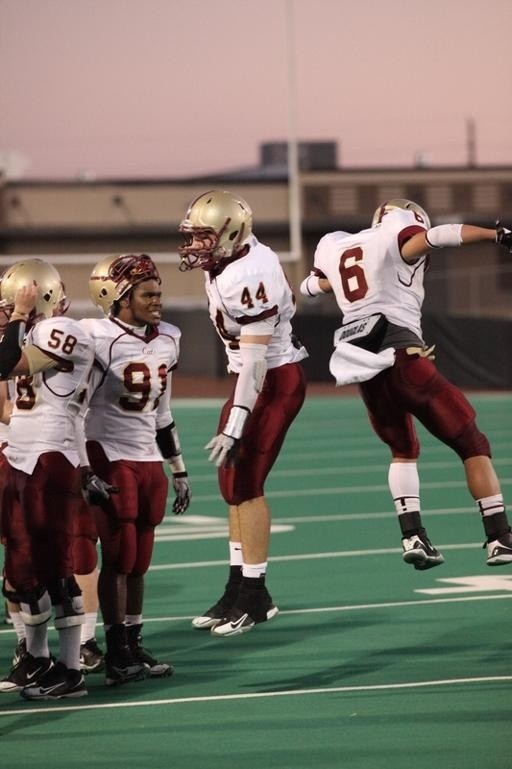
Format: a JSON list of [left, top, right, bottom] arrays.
[[176, 188, 312, 640], [299, 198, 511, 571], [0, 253, 196, 705]]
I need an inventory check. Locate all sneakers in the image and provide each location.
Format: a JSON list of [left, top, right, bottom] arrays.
[[0, 640, 174, 700], [193, 582, 279, 637], [482, 525, 512, 565], [401, 528, 444, 570]]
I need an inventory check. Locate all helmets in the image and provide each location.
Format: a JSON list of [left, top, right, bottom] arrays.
[[88, 253, 161, 317], [0, 259, 67, 335], [177, 190, 253, 272], [371, 198, 431, 228]]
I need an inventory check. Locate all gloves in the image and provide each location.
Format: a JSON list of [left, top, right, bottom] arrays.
[[495, 220, 512, 253], [204, 433, 240, 471], [82, 466, 119, 501], [172, 472, 191, 514]]
